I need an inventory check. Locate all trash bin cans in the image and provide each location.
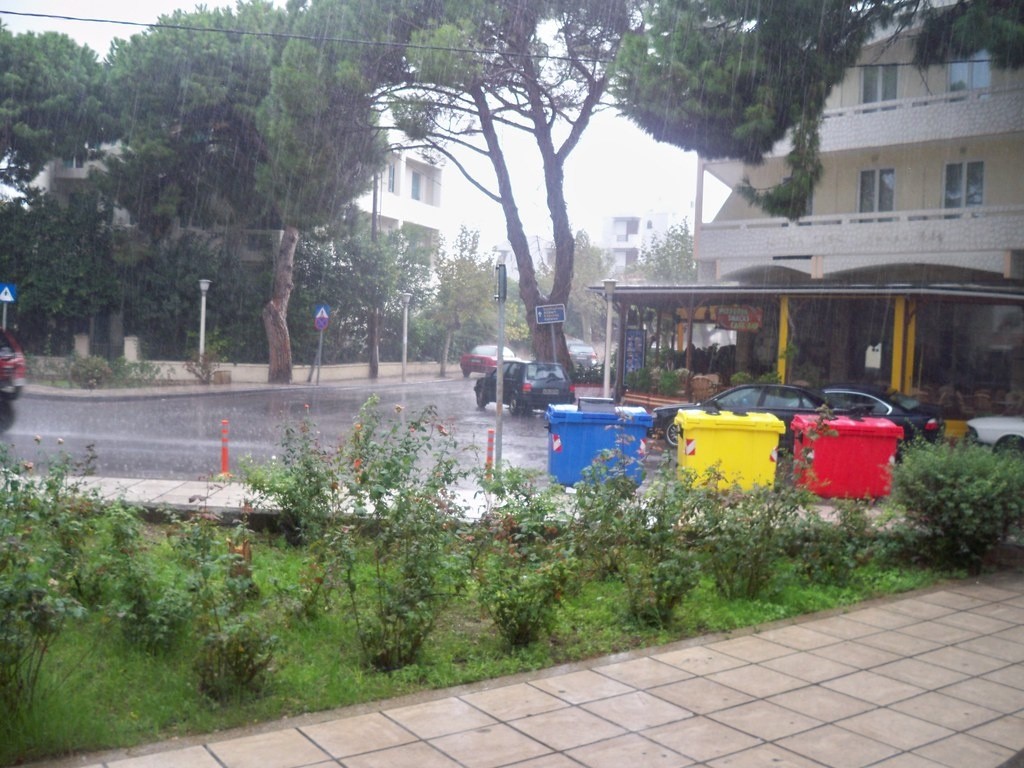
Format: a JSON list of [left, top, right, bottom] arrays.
[[674, 408, 786, 494], [543, 397, 654, 489], [790, 413, 904, 502]]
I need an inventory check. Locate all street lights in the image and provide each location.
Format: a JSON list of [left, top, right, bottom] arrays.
[[399, 291, 413, 384], [198, 279, 213, 365]]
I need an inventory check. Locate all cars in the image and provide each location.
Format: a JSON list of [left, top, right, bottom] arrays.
[[0, 328, 28, 401], [647, 382, 853, 450], [788, 385, 948, 444], [567, 344, 600, 367], [472, 358, 577, 416], [966, 414, 1024, 458], [460, 344, 523, 377]]
